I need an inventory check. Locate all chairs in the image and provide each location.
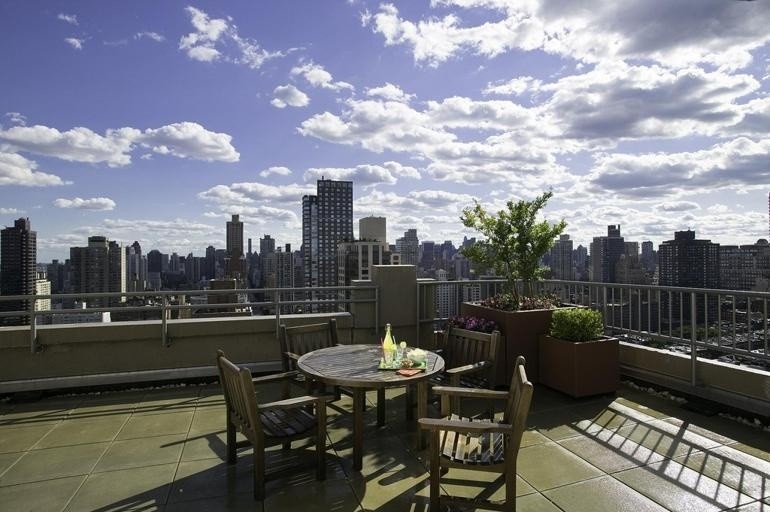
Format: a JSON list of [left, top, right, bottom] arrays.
[[278, 318, 367, 416], [215, 349, 337, 501], [415, 355, 534, 512], [407, 323, 500, 422]]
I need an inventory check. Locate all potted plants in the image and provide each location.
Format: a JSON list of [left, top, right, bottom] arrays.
[[461, 190, 590, 388], [537, 309, 619, 398]]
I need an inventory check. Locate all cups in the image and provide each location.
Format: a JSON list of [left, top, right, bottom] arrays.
[[395, 348, 403, 365]]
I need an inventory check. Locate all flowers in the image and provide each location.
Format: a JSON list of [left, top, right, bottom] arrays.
[[447, 312, 495, 332]]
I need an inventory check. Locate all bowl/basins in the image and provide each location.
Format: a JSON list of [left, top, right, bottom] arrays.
[[407, 354, 426, 364]]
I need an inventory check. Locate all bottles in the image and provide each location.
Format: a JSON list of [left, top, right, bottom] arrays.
[[382, 323, 394, 366]]
